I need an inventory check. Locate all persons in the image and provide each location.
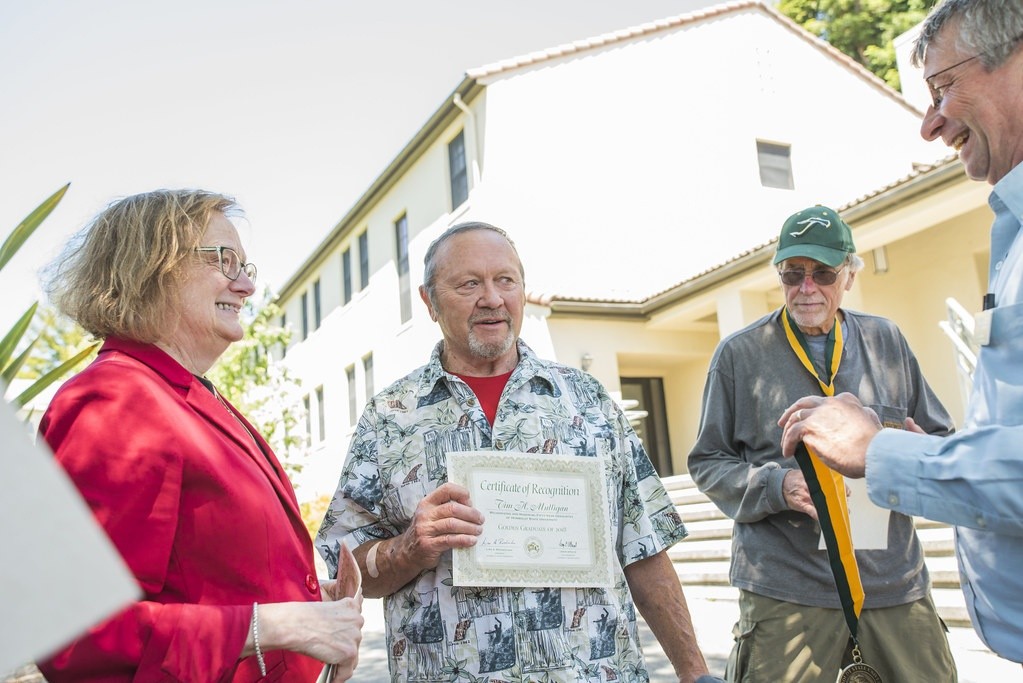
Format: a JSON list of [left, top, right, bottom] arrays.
[[776, 0, 1023, 669], [35, 189, 366, 683], [687, 204, 958, 683], [316, 221, 725, 683]]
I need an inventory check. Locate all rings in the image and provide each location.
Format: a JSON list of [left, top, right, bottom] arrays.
[[798, 410, 802, 421]]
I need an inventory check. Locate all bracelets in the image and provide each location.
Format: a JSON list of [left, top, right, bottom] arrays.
[[695, 676, 723, 683], [366, 540, 383, 578], [253, 602, 266, 677]]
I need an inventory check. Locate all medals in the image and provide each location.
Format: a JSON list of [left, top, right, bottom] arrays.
[[837, 663, 883, 683]]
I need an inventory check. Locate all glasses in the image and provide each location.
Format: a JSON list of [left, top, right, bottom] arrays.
[[779, 263, 846, 285], [924, 35, 1023, 109], [180, 247, 257, 283]]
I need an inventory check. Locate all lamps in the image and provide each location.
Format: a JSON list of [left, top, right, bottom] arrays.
[[582, 352, 594, 372]]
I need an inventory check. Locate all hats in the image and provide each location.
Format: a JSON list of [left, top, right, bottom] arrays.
[[773, 204, 855, 267]]
